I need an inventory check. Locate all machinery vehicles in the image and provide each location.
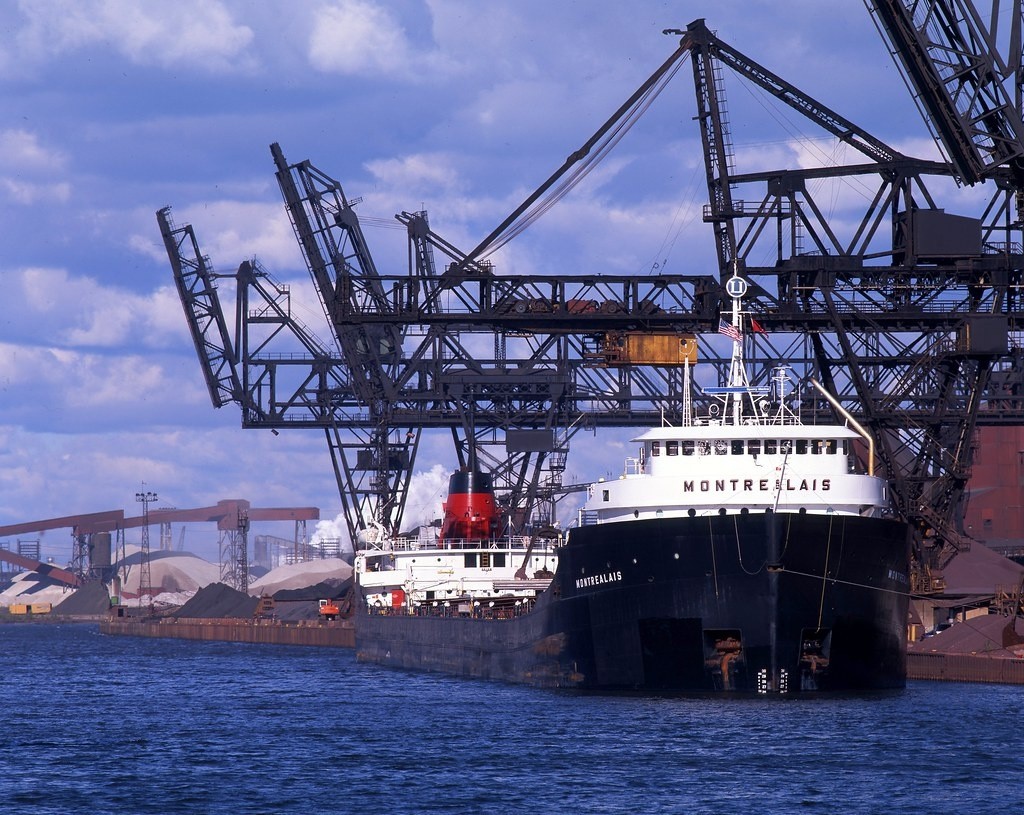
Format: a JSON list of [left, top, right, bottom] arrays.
[[319, 598, 339, 622]]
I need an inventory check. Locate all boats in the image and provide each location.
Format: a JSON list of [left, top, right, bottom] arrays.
[[338, 244, 912, 696]]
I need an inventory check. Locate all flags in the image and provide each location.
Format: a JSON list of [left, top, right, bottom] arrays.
[[718, 317, 743, 342], [750, 317, 769, 339]]
